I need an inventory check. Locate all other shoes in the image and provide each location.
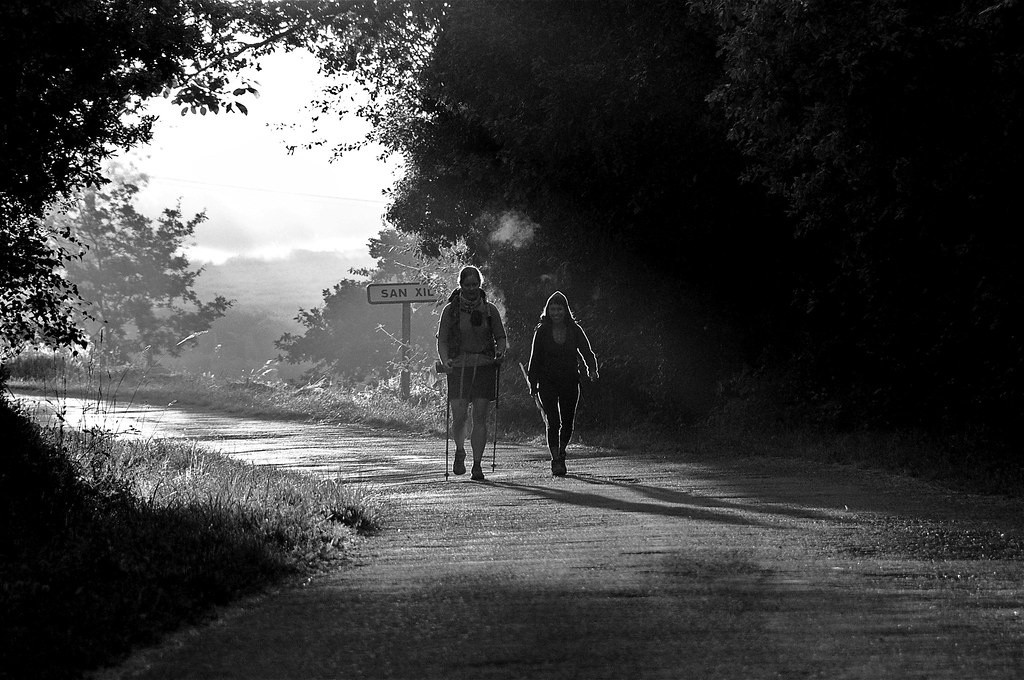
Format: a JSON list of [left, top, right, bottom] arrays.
[[470, 467, 484, 480], [453, 448, 466, 475], [551, 457, 566, 476]]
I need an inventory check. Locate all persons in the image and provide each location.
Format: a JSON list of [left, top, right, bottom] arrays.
[[527, 292, 599, 477], [436, 267, 506, 480]]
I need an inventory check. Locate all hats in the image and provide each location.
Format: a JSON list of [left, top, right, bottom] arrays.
[[548, 291, 568, 305]]
